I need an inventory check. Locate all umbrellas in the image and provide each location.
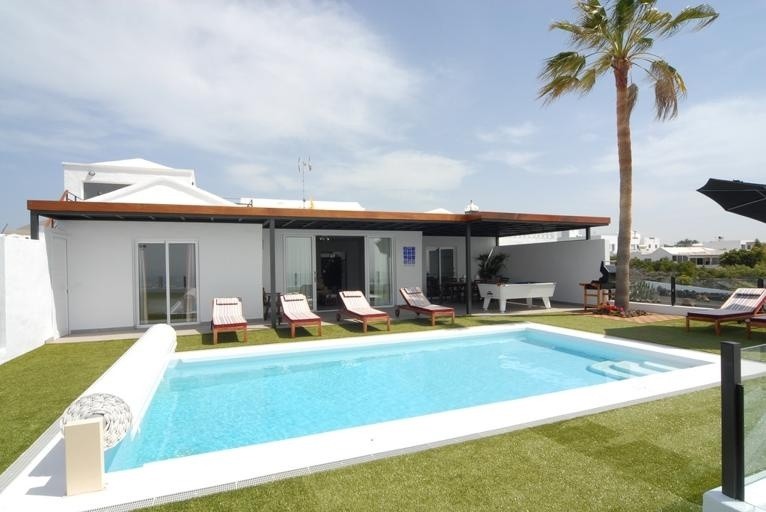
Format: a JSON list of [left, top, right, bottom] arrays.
[[696, 178, 766, 224]]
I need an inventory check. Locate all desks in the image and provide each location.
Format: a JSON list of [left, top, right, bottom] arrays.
[[477, 282, 557, 312]]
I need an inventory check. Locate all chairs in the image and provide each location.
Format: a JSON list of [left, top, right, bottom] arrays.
[[686, 287, 766, 337], [210, 285, 455, 346]]
